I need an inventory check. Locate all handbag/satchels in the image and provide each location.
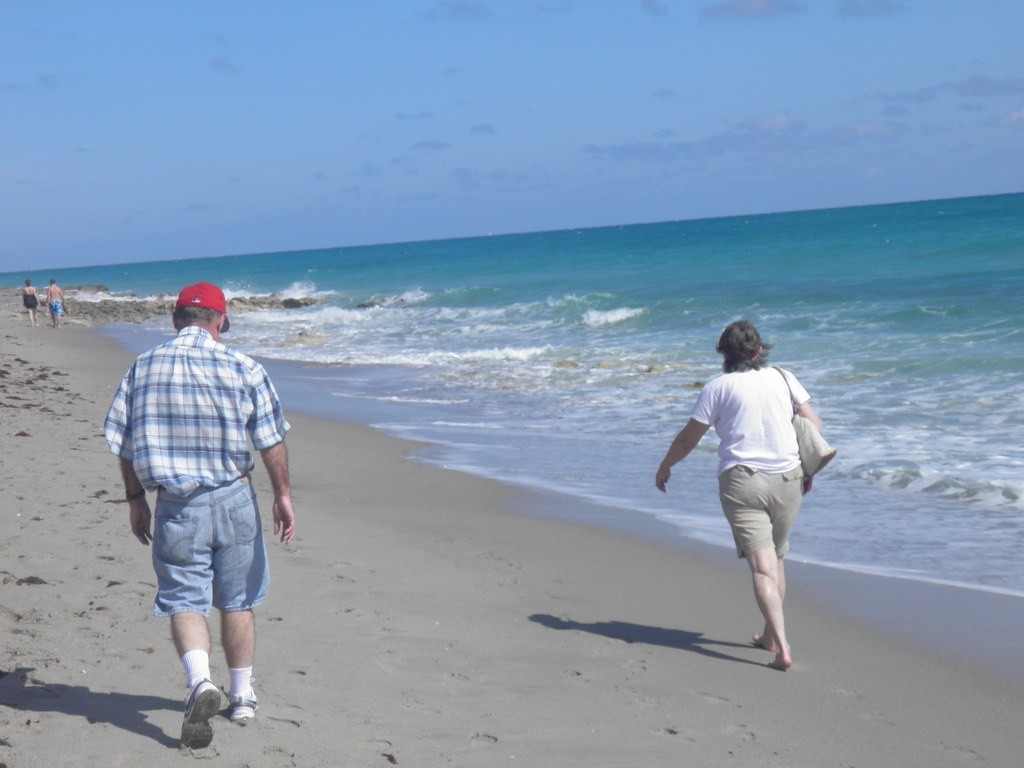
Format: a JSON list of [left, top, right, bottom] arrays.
[[774, 365, 837, 478]]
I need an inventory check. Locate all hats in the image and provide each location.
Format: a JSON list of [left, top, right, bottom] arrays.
[[176, 284, 230, 334]]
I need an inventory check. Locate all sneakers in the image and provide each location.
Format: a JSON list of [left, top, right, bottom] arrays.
[[229, 677, 259, 722], [181, 679, 222, 749]]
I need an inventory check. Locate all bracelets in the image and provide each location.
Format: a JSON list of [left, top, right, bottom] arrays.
[[127, 490, 145, 500]]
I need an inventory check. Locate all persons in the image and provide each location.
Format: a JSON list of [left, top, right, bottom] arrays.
[[656, 320, 822, 669], [22, 280, 41, 327], [104, 282, 296, 747], [47, 279, 64, 328]]
